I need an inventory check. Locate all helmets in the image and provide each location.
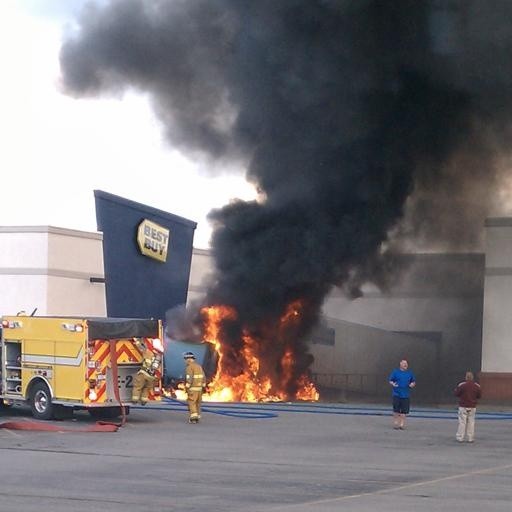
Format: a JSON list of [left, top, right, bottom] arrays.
[[183, 352, 194, 358]]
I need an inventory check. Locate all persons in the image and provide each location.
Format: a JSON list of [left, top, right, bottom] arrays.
[[182, 351, 208, 422], [132, 337, 163, 406], [387, 360, 416, 431], [452, 371, 482, 444]]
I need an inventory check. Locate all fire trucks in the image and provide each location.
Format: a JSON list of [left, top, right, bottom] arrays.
[[0, 309, 164, 422]]
